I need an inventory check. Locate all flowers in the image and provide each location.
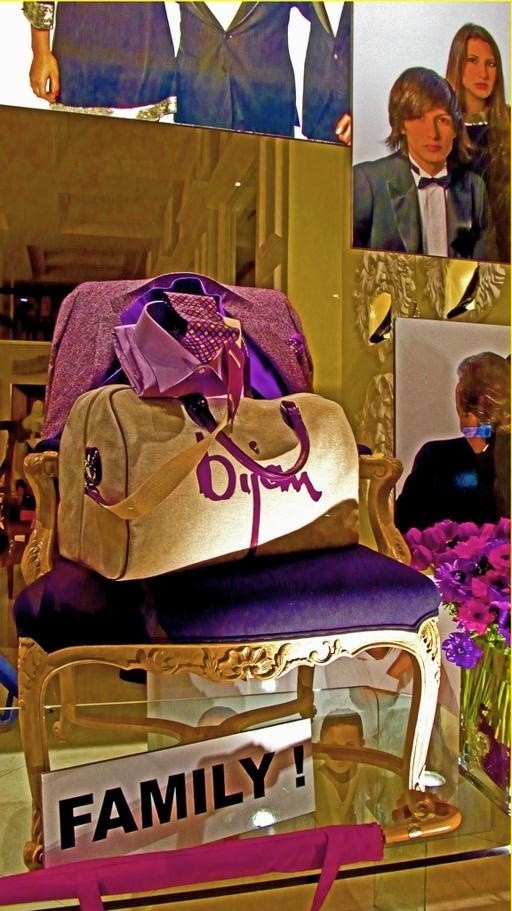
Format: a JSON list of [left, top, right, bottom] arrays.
[[399, 518, 510, 769]]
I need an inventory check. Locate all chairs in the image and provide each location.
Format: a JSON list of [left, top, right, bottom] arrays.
[[11, 278, 465, 871]]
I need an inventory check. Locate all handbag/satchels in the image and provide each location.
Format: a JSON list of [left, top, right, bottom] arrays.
[[53, 396, 363, 584]]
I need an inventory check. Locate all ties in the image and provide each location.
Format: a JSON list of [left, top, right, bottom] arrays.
[[165, 291, 241, 365]]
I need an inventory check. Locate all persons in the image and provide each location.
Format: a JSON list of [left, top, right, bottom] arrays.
[[18, 1, 179, 123], [0, 478, 37, 554], [387, 349, 511, 538], [270, 709, 405, 836], [301, 2, 353, 147], [169, 2, 313, 141], [442, 19, 511, 265], [354, 63, 501, 262]]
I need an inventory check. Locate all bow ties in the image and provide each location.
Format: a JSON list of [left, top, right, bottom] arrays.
[[418, 174, 452, 189]]
